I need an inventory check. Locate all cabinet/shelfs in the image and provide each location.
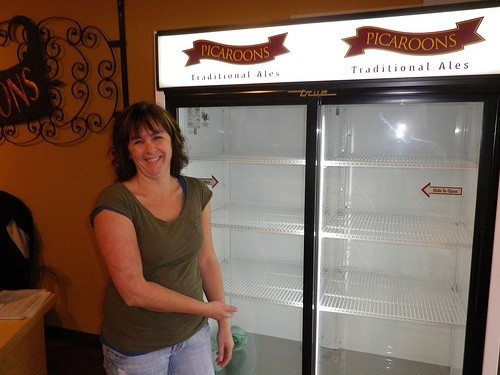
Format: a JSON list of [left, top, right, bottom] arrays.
[[153, 0, 500, 375]]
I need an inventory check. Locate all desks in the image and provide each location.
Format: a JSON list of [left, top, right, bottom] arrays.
[[0, 291, 59, 375]]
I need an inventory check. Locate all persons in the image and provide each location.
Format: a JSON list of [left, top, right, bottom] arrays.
[[90, 101, 239, 375]]
